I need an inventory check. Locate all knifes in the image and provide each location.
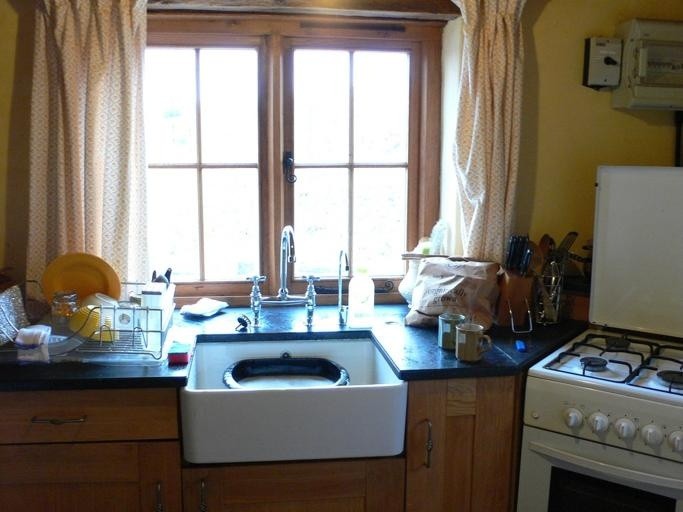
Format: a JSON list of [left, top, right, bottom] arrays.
[[506, 233, 533, 277]]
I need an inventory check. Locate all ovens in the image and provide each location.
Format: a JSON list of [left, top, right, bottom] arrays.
[[515, 377, 682, 511]]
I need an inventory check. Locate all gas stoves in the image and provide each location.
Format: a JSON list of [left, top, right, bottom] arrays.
[[527, 325, 683, 405]]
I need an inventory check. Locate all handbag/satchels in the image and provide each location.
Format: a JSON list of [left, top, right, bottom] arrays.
[[404, 257, 500, 334]]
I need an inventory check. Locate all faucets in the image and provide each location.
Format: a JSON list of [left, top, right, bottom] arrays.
[[278, 223, 297, 300], [337, 249, 349, 325]]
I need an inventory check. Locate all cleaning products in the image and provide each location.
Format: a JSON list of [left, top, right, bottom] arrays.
[[348, 266, 375, 328]]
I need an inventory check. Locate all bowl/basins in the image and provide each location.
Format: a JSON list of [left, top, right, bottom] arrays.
[[80, 291, 119, 317], [67, 305, 119, 342]]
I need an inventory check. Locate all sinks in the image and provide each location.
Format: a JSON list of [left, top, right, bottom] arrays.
[[178, 337, 409, 466]]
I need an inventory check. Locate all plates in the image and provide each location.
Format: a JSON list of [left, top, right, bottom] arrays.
[[41, 253, 120, 317]]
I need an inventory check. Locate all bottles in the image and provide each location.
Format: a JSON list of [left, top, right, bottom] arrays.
[[51, 290, 78, 331], [346, 268, 374, 330]]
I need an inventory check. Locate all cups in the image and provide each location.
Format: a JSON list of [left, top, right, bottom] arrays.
[[437, 313, 465, 351], [454, 324, 491, 363]]
